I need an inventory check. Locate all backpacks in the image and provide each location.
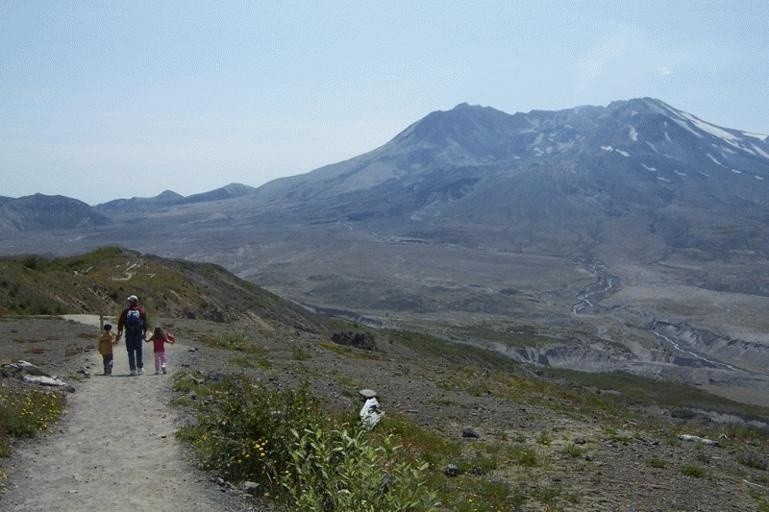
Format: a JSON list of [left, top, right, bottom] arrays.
[[126, 309, 143, 329]]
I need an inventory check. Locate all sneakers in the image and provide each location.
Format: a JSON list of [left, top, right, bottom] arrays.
[[103, 368, 112, 375], [131, 365, 167, 375]]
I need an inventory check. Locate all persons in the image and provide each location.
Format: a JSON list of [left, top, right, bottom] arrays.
[[144, 326, 175, 375], [98, 324, 120, 376], [117, 295, 149, 376]]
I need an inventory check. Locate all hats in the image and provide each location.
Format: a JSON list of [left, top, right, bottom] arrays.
[[128, 295, 138, 304]]
